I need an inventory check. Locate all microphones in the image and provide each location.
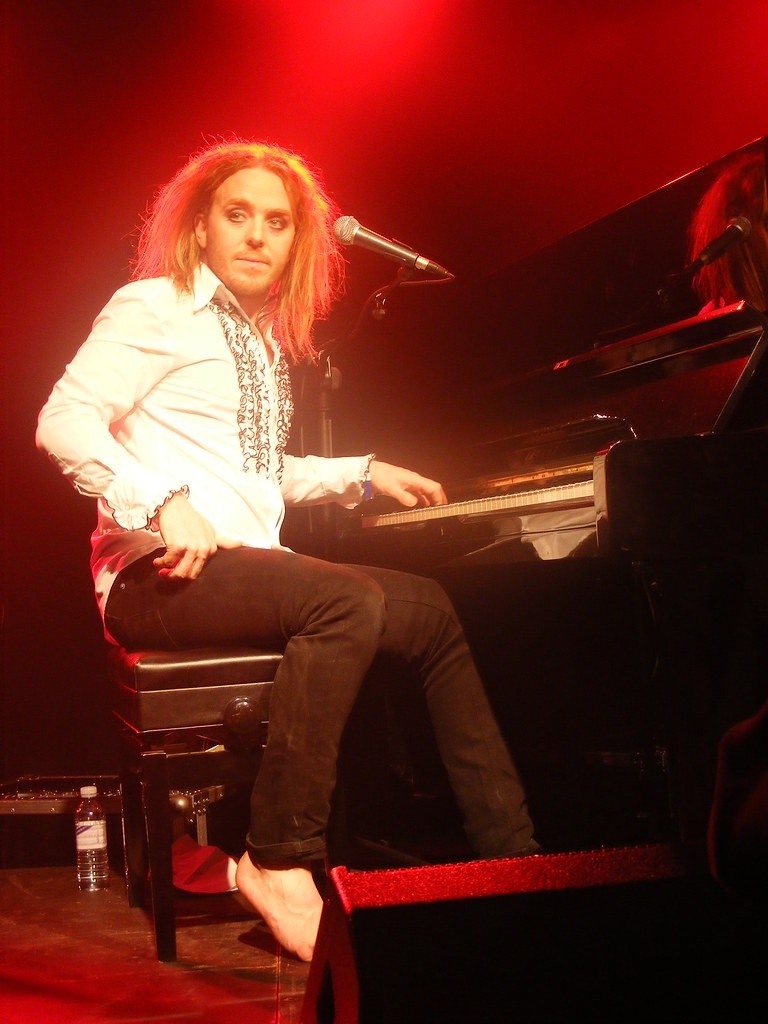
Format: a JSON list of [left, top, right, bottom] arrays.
[[331, 215, 449, 280], [681, 216, 751, 283]]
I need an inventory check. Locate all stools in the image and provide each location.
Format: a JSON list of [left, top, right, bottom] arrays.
[[108, 648, 284, 961]]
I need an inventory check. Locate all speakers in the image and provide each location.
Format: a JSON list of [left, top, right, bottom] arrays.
[[300, 842, 708, 1024]]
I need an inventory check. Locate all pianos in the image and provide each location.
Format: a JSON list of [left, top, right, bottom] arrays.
[[290, 133, 768, 869]]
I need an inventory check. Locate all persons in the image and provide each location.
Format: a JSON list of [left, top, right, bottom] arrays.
[[36, 143, 542, 962], [690, 154, 768, 316]]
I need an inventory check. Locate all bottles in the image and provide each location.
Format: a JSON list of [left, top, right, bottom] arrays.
[[75, 786, 110, 893]]
